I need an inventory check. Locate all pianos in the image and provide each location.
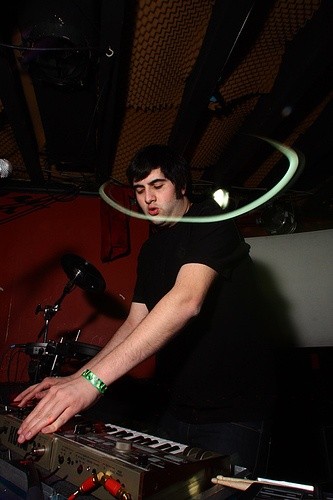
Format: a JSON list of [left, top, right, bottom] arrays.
[[0, 399, 235, 500]]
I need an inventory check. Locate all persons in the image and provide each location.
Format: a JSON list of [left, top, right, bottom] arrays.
[[13, 144, 274, 478]]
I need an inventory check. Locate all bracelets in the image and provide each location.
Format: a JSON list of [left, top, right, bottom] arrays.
[[82, 369, 108, 397]]
